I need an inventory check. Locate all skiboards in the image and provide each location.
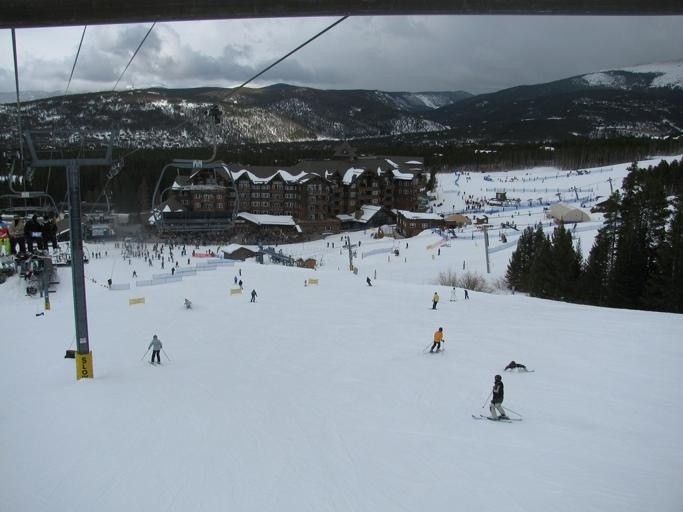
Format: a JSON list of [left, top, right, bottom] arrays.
[[471, 414, 522, 424], [148, 360, 164, 366], [422, 349, 444, 353]]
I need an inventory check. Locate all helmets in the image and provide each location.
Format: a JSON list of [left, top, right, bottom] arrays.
[[494, 375, 500, 381]]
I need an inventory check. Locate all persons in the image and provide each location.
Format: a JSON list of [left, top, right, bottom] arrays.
[[431, 287, 470, 309], [393, 240, 410, 262], [326, 234, 362, 259], [430, 327, 444, 353], [452, 190, 489, 212], [184, 298, 192, 309], [487, 374, 506, 420], [366, 276, 373, 286], [147, 335, 163, 364], [92, 237, 205, 288], [233, 268, 257, 303], [1, 213, 61, 299]]
